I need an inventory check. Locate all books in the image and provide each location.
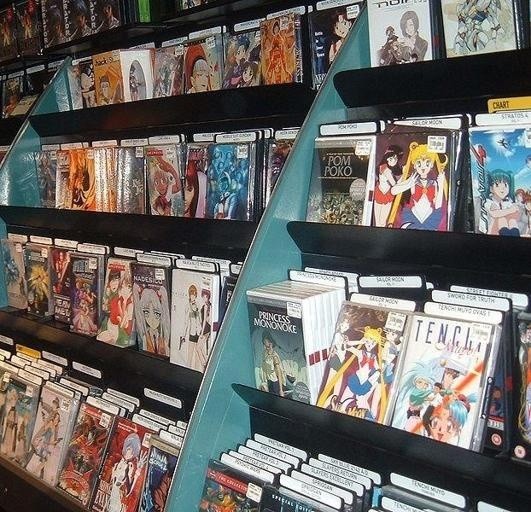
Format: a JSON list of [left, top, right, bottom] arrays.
[[303, 0, 531, 239], [195, 265, 531, 511], [1, 1, 367, 223], [1, 231, 247, 512]]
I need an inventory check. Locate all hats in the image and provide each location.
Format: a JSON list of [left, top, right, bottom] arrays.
[[185, 44, 210, 89]]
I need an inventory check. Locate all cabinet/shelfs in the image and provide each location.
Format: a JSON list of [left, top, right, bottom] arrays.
[[0, 0, 531, 512]]
